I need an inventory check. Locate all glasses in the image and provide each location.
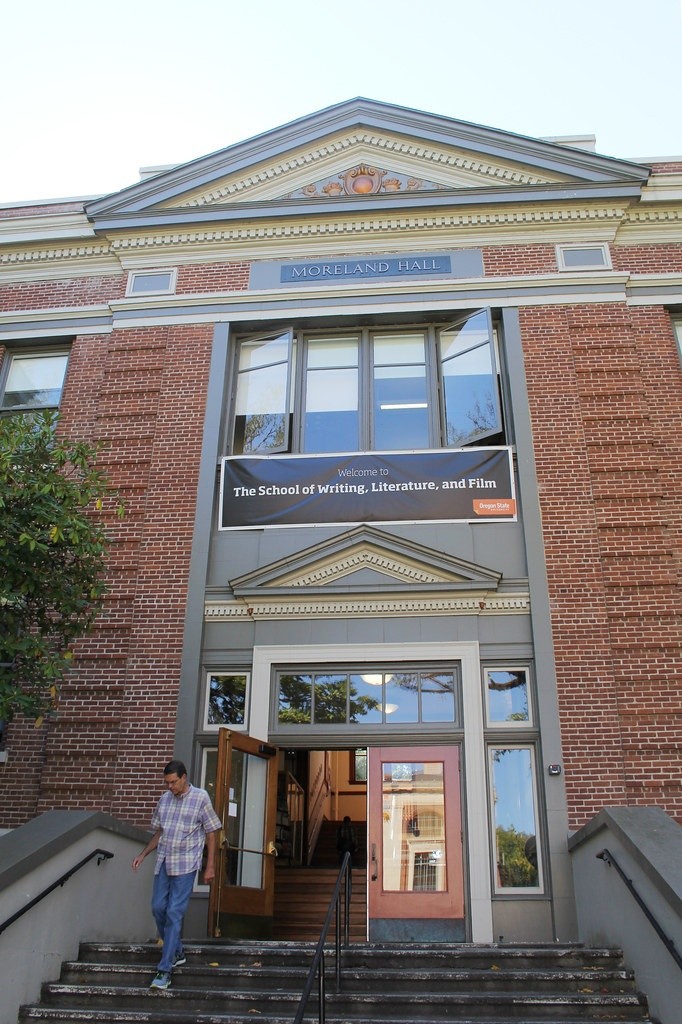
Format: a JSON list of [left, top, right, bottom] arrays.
[[163, 777, 182, 787]]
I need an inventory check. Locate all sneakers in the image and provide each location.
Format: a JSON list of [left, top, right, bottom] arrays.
[[172, 947, 186, 968], [150, 970, 172, 989]]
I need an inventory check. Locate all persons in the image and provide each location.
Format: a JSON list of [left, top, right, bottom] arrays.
[[132, 760, 223, 990], [335, 816, 359, 869]]
